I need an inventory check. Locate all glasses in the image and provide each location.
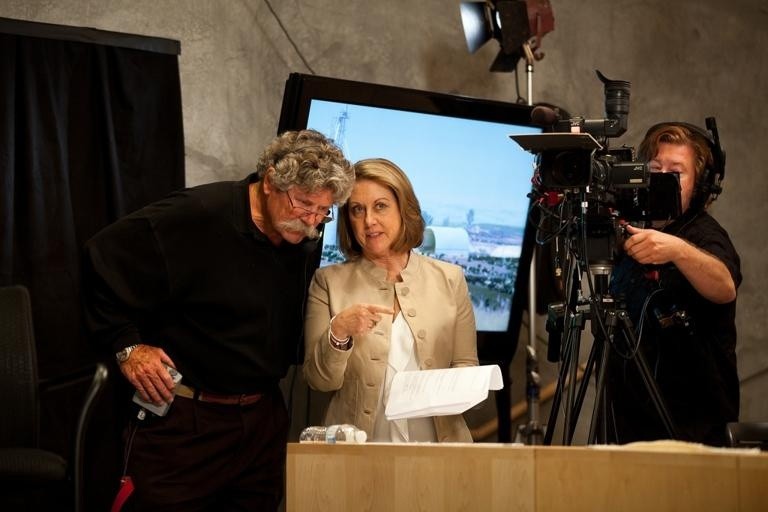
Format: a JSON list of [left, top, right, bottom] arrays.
[[285, 187, 335, 223]]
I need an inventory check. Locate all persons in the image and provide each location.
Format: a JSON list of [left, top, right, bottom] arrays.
[[307, 157, 482, 443], [68, 129, 358, 512], [588, 120, 744, 448]]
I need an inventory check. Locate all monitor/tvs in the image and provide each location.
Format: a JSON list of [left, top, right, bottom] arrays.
[[277, 72, 547, 366]]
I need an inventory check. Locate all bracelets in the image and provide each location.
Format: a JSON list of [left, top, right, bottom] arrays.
[[330, 332, 351, 346]]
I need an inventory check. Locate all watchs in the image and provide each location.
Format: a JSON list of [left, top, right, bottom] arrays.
[[114, 344, 138, 364]]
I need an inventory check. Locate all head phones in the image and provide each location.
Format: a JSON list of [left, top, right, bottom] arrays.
[[645, 122, 725, 213]]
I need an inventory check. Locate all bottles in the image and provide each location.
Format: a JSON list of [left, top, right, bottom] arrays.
[[300, 424, 368, 443]]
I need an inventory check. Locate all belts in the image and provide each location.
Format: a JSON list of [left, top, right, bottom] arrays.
[[175, 385, 264, 405]]
